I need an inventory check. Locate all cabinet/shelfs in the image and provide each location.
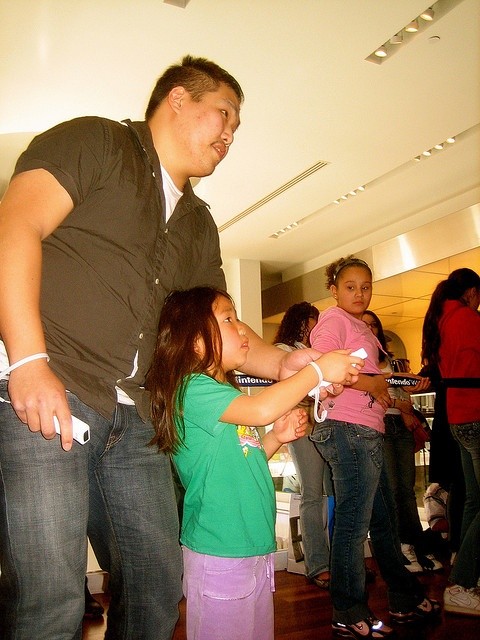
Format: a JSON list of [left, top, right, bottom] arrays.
[[287, 494, 336, 575]]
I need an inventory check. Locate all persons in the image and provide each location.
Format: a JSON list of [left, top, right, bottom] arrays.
[[440, 266, 480, 618], [146, 284, 364, 639], [310, 259, 443, 638], [362, 309, 446, 576], [420, 276, 461, 580], [1, 58, 346, 640], [273, 302, 334, 589]]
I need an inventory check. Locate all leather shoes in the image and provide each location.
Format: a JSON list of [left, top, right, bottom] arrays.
[[313, 572, 331, 587]]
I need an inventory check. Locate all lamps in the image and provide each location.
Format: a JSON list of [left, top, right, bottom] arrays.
[[435, 143, 443, 150], [341, 195, 348, 200], [446, 136, 455, 144], [334, 199, 340, 205], [404, 21, 418, 33], [414, 155, 421, 161], [389, 34, 402, 45], [358, 185, 366, 191], [374, 45, 387, 57], [423, 149, 431, 156], [350, 190, 357, 196], [419, 7, 434, 21]]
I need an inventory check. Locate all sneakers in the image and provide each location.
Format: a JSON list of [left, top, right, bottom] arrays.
[[399, 542, 424, 573], [443, 581, 480, 617], [389, 595, 442, 625], [82, 583, 105, 614], [419, 552, 444, 572], [332, 612, 397, 639]]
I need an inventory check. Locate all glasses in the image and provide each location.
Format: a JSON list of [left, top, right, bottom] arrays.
[[364, 321, 378, 328], [308, 314, 318, 320]]
